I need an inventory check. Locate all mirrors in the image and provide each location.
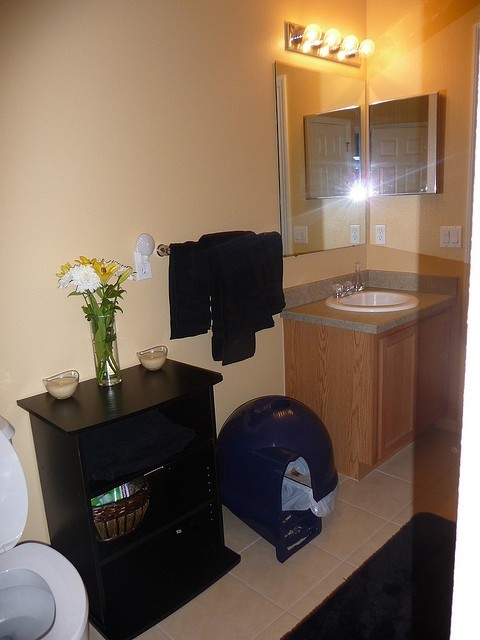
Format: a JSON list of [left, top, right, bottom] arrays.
[[273, 62, 371, 258], [369, 91, 443, 198], [303, 106, 360, 200]]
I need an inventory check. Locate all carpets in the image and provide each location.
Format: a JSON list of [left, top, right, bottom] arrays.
[[280, 510, 458, 639]]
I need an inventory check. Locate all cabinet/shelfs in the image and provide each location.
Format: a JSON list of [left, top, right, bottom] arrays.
[[15, 358, 242, 639]]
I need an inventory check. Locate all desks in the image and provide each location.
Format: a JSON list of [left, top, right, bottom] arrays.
[[282, 294, 459, 483]]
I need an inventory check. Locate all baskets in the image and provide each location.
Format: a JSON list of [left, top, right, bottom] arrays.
[[90, 476, 152, 542]]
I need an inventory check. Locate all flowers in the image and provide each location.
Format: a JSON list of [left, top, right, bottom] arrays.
[[56, 254, 139, 384]]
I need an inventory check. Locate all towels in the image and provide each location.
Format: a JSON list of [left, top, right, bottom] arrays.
[[167, 239, 212, 341], [257, 229, 286, 317], [201, 229, 277, 367]]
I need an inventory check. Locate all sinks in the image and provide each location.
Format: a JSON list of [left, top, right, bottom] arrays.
[[326, 289, 419, 312]]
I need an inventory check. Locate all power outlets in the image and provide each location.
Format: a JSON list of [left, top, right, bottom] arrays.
[[134, 257, 154, 282], [373, 224, 387, 248], [349, 225, 360, 246]]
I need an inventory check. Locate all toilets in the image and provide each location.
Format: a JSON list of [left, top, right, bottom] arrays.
[[0, 417, 89, 640]]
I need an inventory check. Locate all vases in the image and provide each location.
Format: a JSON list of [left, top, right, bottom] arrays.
[[89, 312, 123, 389]]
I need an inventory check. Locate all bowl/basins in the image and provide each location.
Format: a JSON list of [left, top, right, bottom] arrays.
[[136, 345, 168, 371], [43, 370, 79, 400]]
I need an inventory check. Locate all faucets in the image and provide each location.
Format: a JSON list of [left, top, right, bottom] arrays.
[[329, 276, 354, 297]]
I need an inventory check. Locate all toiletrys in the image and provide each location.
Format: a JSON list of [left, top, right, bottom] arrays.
[[355, 261, 365, 291]]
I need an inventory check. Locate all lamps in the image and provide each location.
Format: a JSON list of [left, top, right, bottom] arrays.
[[330, 34, 358, 56], [347, 38, 376, 60], [290, 23, 321, 46], [313, 28, 341, 50]]
